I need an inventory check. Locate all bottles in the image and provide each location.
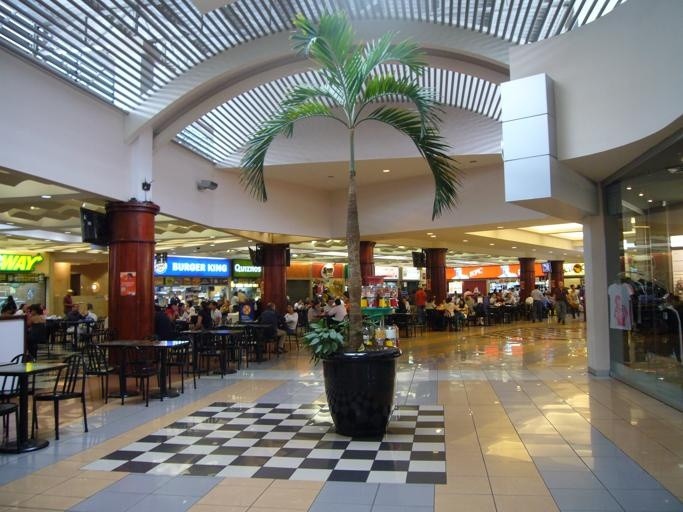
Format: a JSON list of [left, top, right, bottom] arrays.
[[362, 324, 400, 348]]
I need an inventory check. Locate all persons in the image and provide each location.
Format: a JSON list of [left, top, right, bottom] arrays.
[[415, 286, 485, 331], [489, 287, 521, 325], [361, 293, 411, 322], [255, 299, 299, 354], [155, 287, 250, 352], [61, 290, 97, 352], [2, 295, 49, 363], [530, 280, 584, 326], [294, 289, 349, 333]]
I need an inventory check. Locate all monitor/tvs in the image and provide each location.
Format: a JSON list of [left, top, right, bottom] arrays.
[[542, 262, 553, 274], [412, 252, 427, 267], [249, 248, 257, 266], [286, 249, 290, 267], [80, 207, 109, 247]]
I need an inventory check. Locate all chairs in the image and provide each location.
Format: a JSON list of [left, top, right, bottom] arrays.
[[2, 317, 299, 440]]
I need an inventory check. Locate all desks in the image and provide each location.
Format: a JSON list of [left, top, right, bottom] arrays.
[[0, 363, 69, 454]]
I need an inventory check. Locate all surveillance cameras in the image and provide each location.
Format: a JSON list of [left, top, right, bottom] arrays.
[[198, 179, 218, 190]]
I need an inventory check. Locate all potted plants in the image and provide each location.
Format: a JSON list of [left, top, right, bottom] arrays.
[[236, 6, 467, 437]]
